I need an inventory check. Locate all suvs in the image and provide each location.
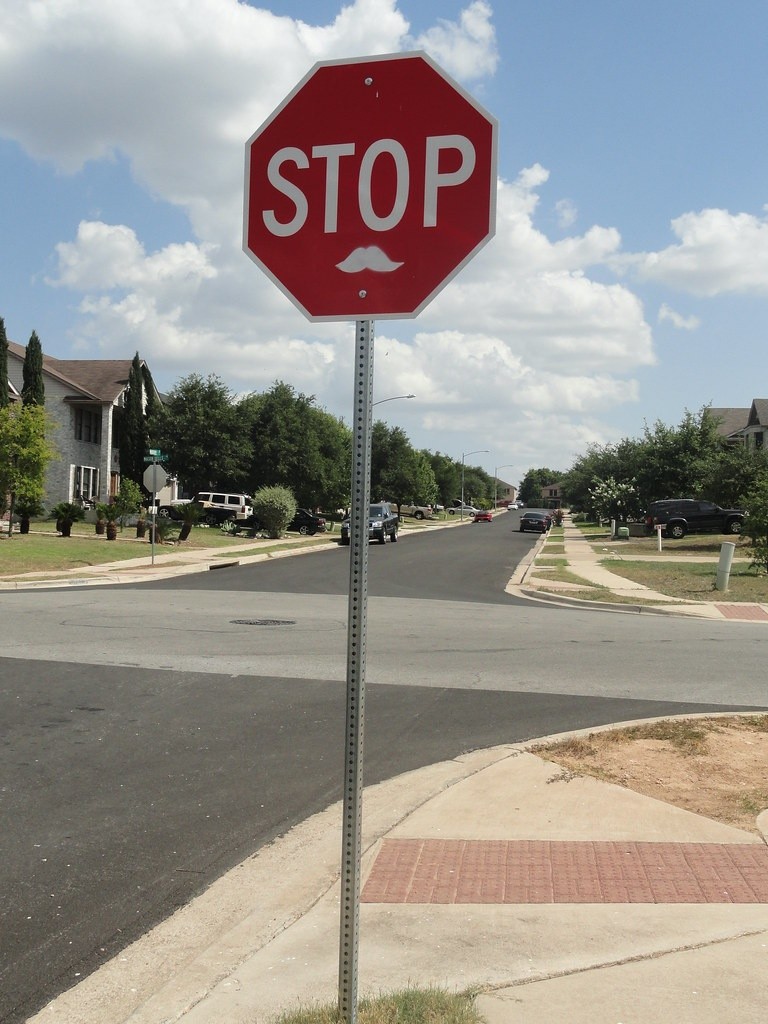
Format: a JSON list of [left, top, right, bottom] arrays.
[[644, 499, 747, 539], [193, 492, 254, 521]]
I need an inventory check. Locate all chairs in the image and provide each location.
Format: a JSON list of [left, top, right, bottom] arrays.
[[80, 495, 95, 511]]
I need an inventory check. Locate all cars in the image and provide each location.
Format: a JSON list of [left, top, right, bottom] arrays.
[[157, 501, 237, 528], [447, 505, 480, 517], [514, 500, 524, 508], [506, 503, 517, 510], [474, 510, 493, 523], [339, 504, 399, 544], [386, 499, 433, 520], [520, 513, 548, 533], [286, 506, 326, 536]]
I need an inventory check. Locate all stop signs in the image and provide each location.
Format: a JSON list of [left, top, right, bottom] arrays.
[[241, 55, 499, 317]]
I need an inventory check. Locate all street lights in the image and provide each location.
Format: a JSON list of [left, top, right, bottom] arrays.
[[493, 463, 515, 510], [461, 449, 490, 506]]
